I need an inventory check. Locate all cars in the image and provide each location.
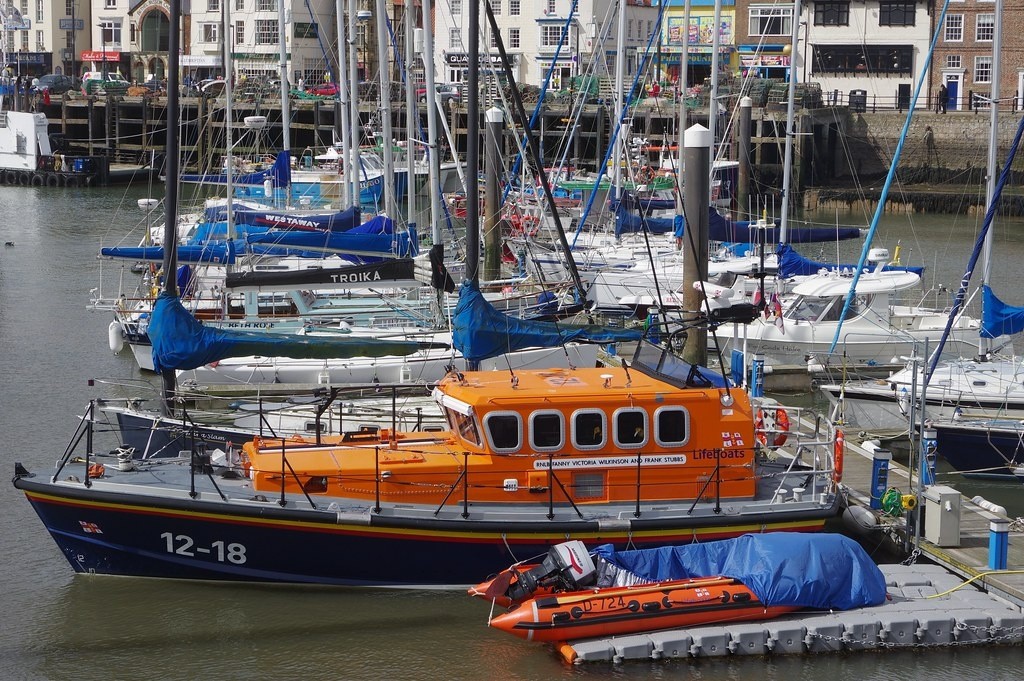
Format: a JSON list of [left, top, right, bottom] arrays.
[[142, 79, 166, 91], [436, 85, 463, 106], [269, 79, 290, 90], [305, 83, 340, 96], [413, 82, 427, 104], [30, 74, 77, 95]]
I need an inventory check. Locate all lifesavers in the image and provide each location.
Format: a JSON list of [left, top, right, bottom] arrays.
[[833, 429, 844, 483], [520, 216, 540, 236], [4, 171, 80, 188], [638, 166, 655, 184], [756, 405, 789, 451]]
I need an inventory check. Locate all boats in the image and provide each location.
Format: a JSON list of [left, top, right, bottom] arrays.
[[467, 527, 883, 642]]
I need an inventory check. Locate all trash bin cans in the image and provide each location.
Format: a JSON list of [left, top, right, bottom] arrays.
[[848, 89, 867, 113]]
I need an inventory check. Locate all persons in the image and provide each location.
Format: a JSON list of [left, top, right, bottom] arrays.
[[297, 76, 304, 91], [940, 84, 949, 114]]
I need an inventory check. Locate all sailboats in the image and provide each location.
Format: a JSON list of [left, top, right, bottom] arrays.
[[0, 2, 1024, 589]]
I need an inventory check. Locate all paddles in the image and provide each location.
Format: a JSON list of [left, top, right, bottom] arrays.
[[557, 579, 734, 604]]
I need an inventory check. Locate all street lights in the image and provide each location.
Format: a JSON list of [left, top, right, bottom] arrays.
[[230, 23, 235, 75], [800, 21, 808, 82], [570, 23, 580, 76], [96, 23, 106, 86], [357, 9, 373, 82]]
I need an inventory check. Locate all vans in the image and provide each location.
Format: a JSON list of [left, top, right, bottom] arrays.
[[82, 71, 131, 89]]
